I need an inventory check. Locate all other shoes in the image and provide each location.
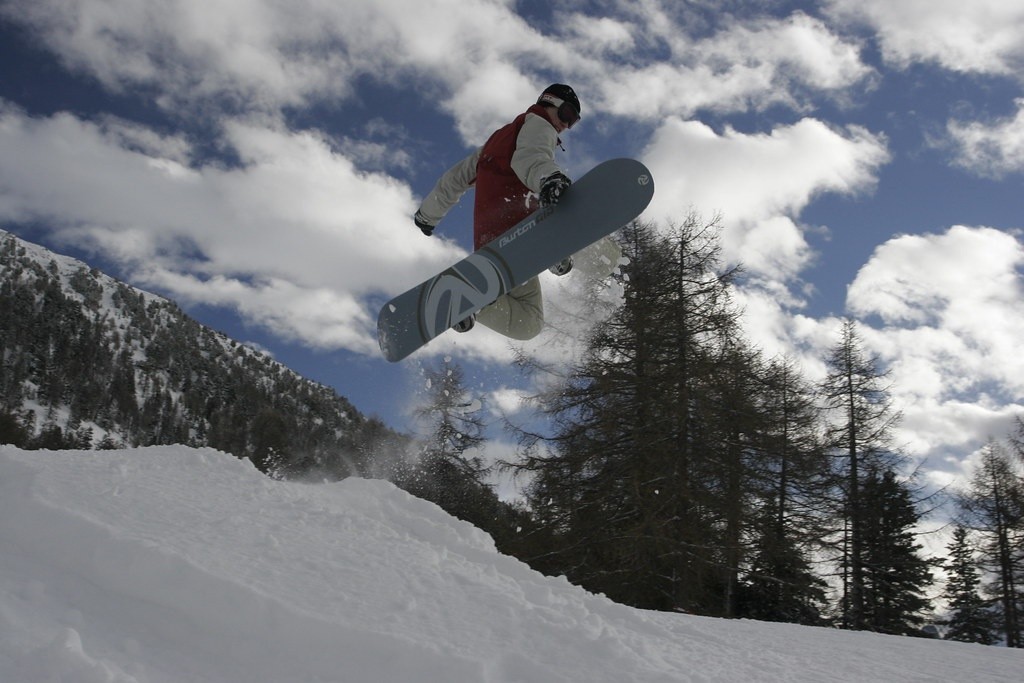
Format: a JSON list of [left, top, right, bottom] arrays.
[[548, 256, 574, 276], [452, 312, 477, 333]]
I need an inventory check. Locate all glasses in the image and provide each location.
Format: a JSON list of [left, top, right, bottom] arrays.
[[538, 92, 582, 130]]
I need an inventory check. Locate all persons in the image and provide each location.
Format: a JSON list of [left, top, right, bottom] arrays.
[[413, 84, 581, 341]]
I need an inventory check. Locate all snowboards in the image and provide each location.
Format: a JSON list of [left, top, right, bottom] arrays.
[[376, 158, 655, 365]]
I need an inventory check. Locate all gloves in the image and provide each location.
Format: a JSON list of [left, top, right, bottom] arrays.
[[414, 208, 436, 236], [536, 170, 572, 209]]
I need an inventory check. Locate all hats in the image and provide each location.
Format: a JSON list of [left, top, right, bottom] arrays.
[[537, 83, 581, 113]]
